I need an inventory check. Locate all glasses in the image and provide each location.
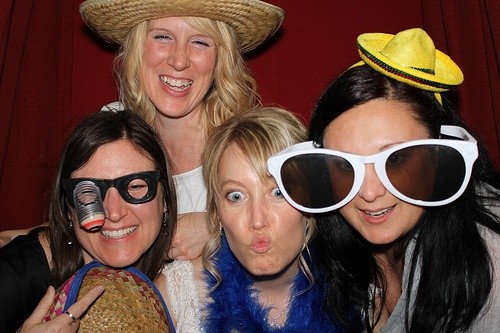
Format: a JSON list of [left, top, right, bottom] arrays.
[[267, 125, 479, 213], [64, 170, 161, 232]]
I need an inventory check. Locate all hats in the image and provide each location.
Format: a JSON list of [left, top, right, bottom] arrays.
[[355, 27, 465, 92], [79, 0, 286, 54], [42, 261, 176, 333]]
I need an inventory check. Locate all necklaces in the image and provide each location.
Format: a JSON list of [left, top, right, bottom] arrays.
[[263, 299, 293, 326]]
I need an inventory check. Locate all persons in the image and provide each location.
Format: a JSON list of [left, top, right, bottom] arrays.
[[78, 0, 287, 216], [267, 27, 500, 333], [0, 109, 178, 332], [153, 105, 368, 333]]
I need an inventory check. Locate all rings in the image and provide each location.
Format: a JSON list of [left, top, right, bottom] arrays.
[[64, 311, 75, 321]]
